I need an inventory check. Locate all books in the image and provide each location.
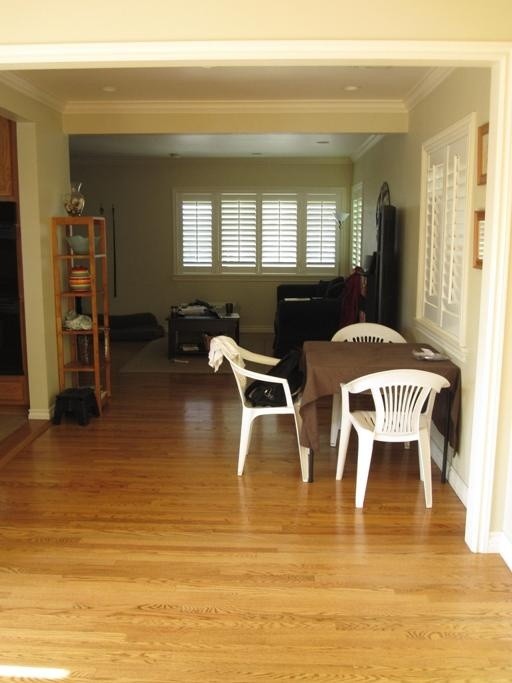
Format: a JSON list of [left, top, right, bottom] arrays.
[[182, 343, 200, 352], [218, 310, 241, 318]]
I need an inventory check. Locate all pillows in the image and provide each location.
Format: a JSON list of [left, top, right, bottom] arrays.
[[315, 276, 344, 297]]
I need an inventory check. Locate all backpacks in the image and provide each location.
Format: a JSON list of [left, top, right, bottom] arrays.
[[245, 348, 306, 408]]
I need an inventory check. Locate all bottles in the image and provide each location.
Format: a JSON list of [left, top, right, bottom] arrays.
[[68, 266, 91, 293], [64, 182, 87, 217]]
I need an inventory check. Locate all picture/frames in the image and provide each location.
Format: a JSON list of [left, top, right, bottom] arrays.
[[472, 210, 485, 270], [476, 122, 488, 185]]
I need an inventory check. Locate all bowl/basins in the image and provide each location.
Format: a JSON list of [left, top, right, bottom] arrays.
[[67, 234, 100, 254]]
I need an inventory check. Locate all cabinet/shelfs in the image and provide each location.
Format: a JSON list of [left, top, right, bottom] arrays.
[[50, 216, 112, 417]]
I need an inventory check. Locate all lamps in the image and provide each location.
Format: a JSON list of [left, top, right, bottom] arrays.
[[333, 212, 350, 278]]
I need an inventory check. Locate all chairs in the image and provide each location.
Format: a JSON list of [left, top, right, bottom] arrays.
[[335, 369, 451, 509], [272, 283, 343, 358], [329, 321, 411, 450], [220, 347, 310, 484]]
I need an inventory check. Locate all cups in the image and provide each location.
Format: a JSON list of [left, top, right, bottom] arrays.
[[225, 303, 233, 315]]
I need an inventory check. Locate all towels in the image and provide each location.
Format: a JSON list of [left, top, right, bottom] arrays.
[[208, 334, 240, 372]]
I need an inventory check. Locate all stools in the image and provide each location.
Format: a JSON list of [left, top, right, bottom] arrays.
[[53, 388, 101, 426]]
